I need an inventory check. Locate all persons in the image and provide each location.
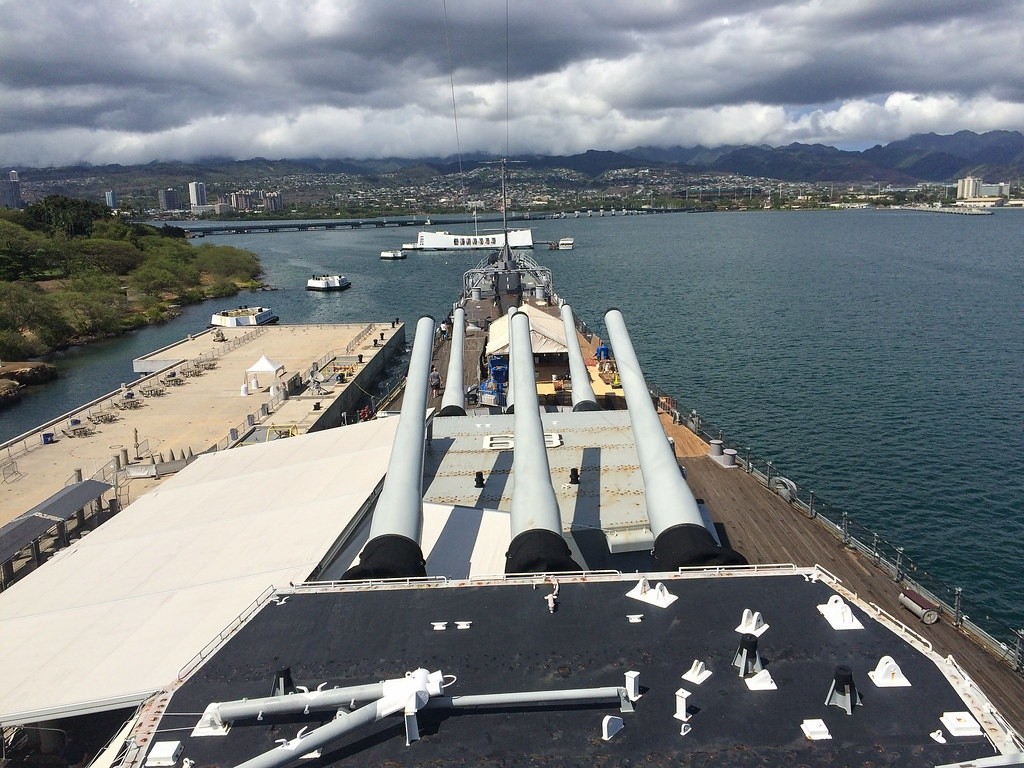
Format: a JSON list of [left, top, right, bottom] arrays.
[[430, 364, 442, 399]]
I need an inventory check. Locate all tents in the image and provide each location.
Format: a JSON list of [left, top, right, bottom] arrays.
[[245, 354, 284, 391], [486, 302, 569, 377]]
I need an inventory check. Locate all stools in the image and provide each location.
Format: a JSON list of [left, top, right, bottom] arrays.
[[160, 375, 185, 387], [195, 362, 217, 370], [114, 396, 143, 410], [87, 412, 118, 425], [139, 384, 164, 397], [62, 423, 96, 439], [180, 368, 201, 378]]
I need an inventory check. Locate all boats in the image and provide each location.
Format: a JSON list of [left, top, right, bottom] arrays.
[[560, 236, 575, 250], [207, 305, 280, 326], [305, 273, 353, 291], [401, 206, 534, 251], [378, 249, 408, 260]]
